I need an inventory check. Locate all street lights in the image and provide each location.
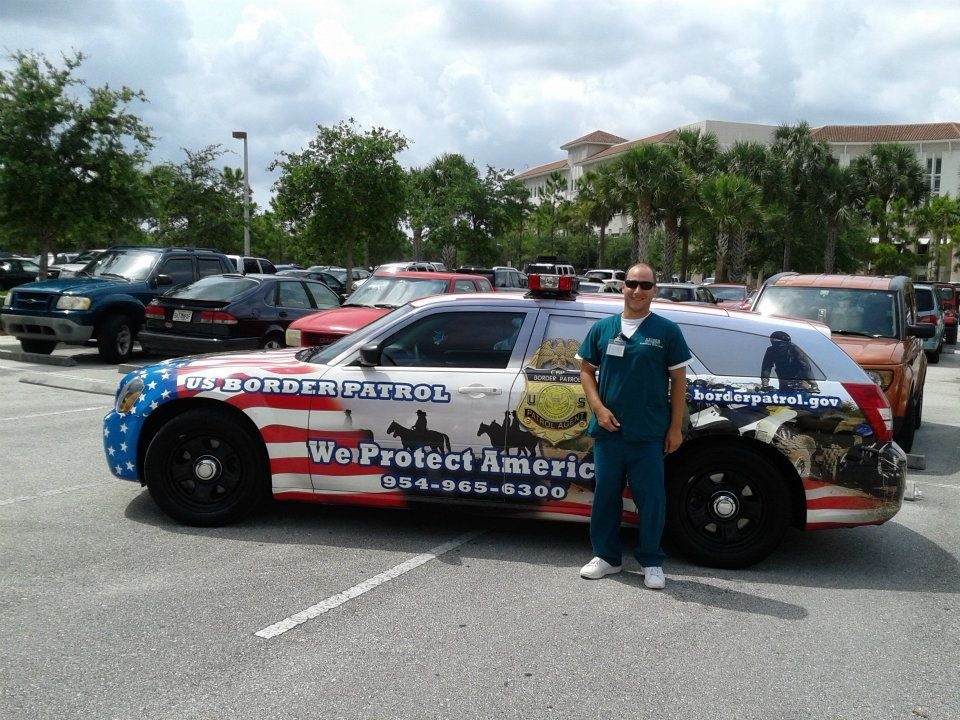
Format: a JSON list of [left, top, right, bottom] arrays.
[[232, 130, 251, 257]]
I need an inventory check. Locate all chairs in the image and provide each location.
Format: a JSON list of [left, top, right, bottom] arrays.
[[863, 299, 889, 329]]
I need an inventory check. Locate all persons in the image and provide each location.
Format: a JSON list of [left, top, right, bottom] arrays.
[[574, 261, 693, 589]]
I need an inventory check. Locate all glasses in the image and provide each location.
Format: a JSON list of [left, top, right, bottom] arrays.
[[626, 280, 655, 290]]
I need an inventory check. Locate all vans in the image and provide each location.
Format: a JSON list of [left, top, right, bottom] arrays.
[[523, 261, 577, 278]]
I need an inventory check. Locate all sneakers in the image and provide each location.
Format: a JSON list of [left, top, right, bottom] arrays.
[[643, 565, 665, 588], [580, 556, 622, 579]]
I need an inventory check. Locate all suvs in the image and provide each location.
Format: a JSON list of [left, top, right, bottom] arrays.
[[453, 264, 529, 289], [350, 260, 447, 291], [0, 244, 278, 364], [101, 274, 906, 572], [749, 272, 960, 453]]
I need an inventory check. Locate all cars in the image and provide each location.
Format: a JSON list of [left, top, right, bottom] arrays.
[[577, 268, 628, 294], [285, 270, 497, 347], [652, 282, 759, 312], [135, 264, 372, 358]]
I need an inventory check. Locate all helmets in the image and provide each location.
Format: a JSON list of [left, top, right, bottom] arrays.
[[770, 331, 791, 343]]
